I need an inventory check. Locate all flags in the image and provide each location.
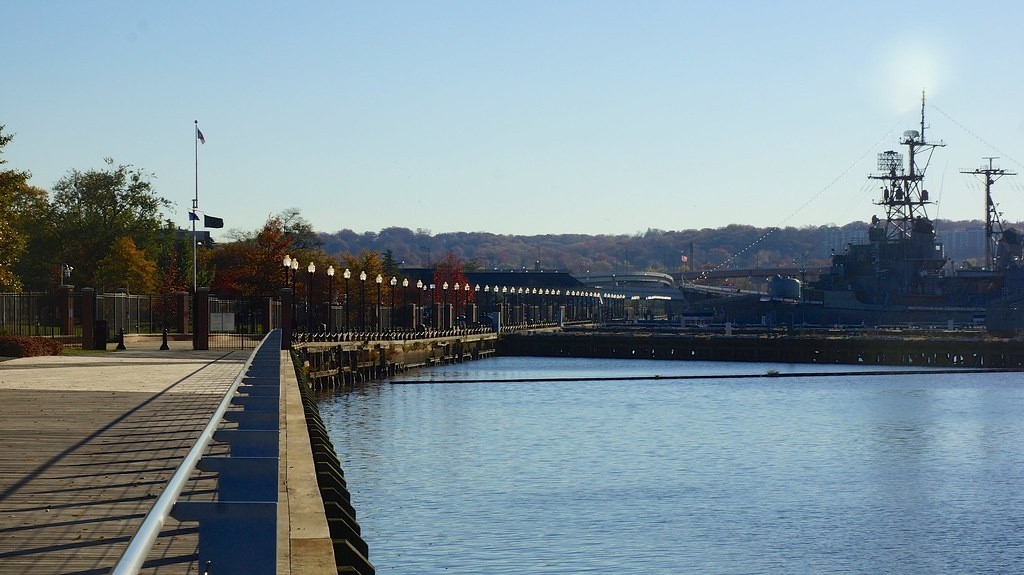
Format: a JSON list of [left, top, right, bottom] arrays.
[[198, 129, 205, 144], [682, 255, 687, 263]]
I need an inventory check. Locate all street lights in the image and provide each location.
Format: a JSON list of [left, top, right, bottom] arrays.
[[429, 280, 436, 331], [375, 274, 383, 333], [484, 284, 490, 327], [494, 284, 499, 313], [61, 263, 73, 284], [416, 280, 423, 333], [454, 282, 460, 326], [475, 284, 480, 328], [308, 261, 316, 333], [344, 268, 351, 332], [291, 257, 299, 329], [283, 255, 292, 288], [464, 283, 470, 329], [359, 270, 367, 331], [391, 276, 397, 333], [327, 265, 335, 334], [442, 281, 448, 331], [402, 277, 409, 333], [510, 286, 640, 326], [502, 286, 507, 326]]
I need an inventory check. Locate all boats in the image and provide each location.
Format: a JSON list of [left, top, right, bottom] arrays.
[[676, 86, 1023, 336]]
